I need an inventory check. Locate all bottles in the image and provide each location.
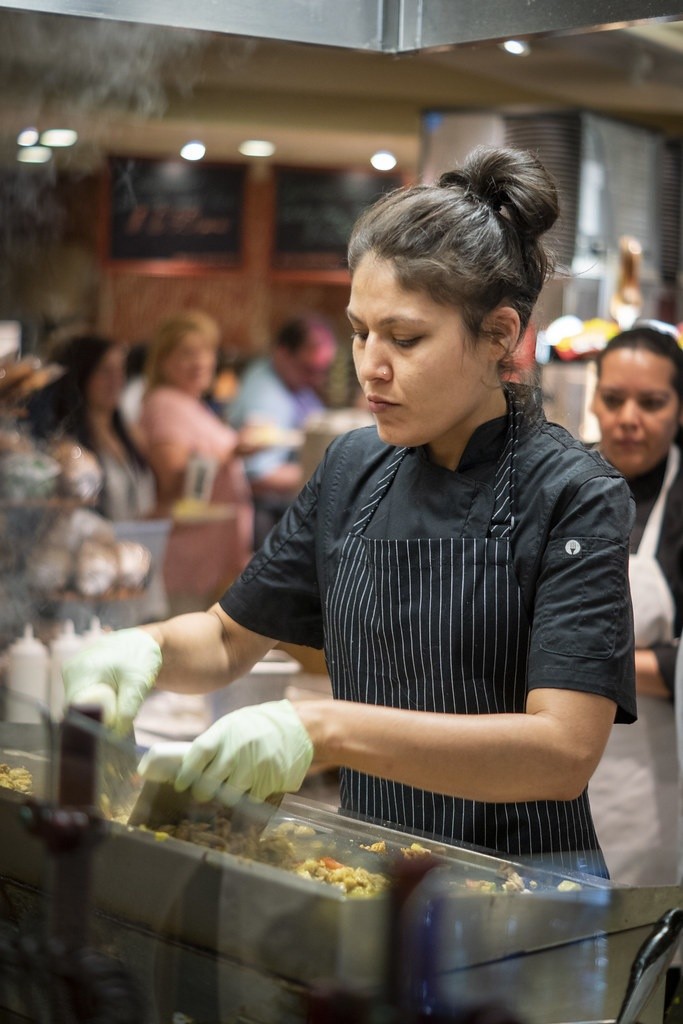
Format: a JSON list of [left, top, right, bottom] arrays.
[[48, 618, 106, 723], [4, 624, 49, 722]]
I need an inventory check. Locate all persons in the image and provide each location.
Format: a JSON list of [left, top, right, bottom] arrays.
[[229, 320, 335, 549], [582, 328, 683, 887], [138, 311, 248, 612], [53, 335, 157, 520], [62, 145, 638, 873]]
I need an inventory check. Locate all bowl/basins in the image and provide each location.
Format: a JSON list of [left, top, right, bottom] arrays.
[[200, 661, 302, 727]]
